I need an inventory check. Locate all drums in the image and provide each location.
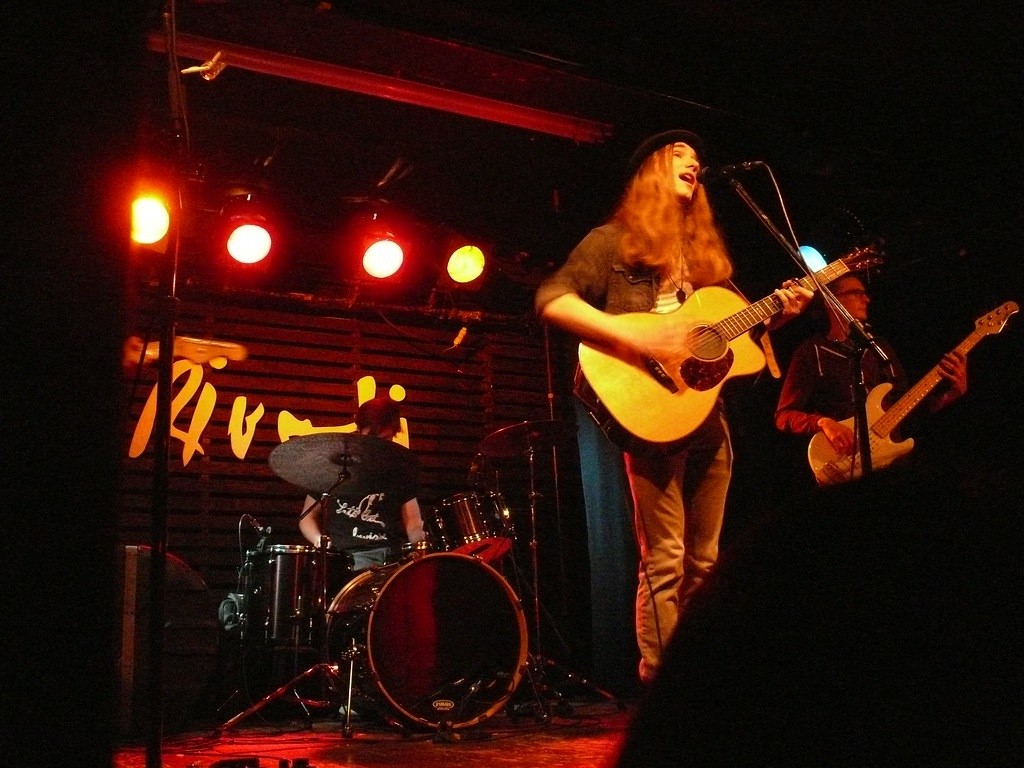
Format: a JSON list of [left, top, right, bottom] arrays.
[[422, 490, 513, 562], [239, 544, 355, 649], [322, 552, 529, 734]]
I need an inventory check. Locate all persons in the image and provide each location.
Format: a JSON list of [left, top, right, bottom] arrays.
[[534, 130, 813, 679], [300, 398, 425, 570], [773, 270, 968, 486]]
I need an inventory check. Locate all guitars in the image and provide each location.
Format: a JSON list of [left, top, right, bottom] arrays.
[[576, 243, 887, 456], [808, 300, 1020, 485]]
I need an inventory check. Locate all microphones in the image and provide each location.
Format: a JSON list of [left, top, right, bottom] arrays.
[[695, 160, 763, 185], [247, 514, 272, 544]]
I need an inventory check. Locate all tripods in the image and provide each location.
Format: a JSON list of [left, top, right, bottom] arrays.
[[523, 435, 623, 720], [209, 454, 406, 737]]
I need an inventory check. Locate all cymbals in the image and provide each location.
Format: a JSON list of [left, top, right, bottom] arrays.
[[268, 433, 420, 495], [479, 418, 579, 458]]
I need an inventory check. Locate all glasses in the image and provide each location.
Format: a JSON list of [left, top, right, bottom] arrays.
[[839, 289, 867, 298]]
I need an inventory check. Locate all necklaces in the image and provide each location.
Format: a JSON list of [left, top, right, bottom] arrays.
[[668, 248, 685, 303]]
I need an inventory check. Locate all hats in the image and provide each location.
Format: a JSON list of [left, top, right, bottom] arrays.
[[620, 128, 704, 185]]
